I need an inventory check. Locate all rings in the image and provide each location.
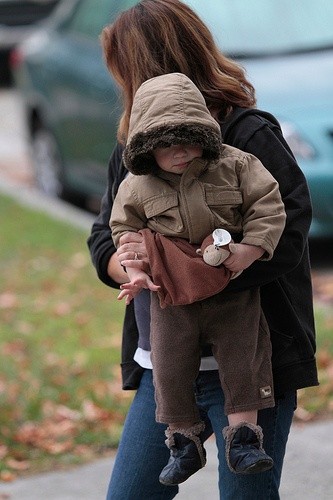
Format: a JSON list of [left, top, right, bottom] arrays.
[[135, 252, 139, 260]]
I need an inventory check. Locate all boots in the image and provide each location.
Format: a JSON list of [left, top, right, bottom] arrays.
[[222, 421, 276, 473], [159, 421, 210, 485]]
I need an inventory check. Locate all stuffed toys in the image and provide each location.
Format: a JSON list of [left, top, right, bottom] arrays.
[[196, 228, 235, 268]]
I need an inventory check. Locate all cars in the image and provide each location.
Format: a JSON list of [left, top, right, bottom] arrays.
[[11, 1, 332, 257]]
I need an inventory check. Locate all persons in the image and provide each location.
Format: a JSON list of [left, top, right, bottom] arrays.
[[86, 0, 321, 499], [107, 72, 287, 487]]
